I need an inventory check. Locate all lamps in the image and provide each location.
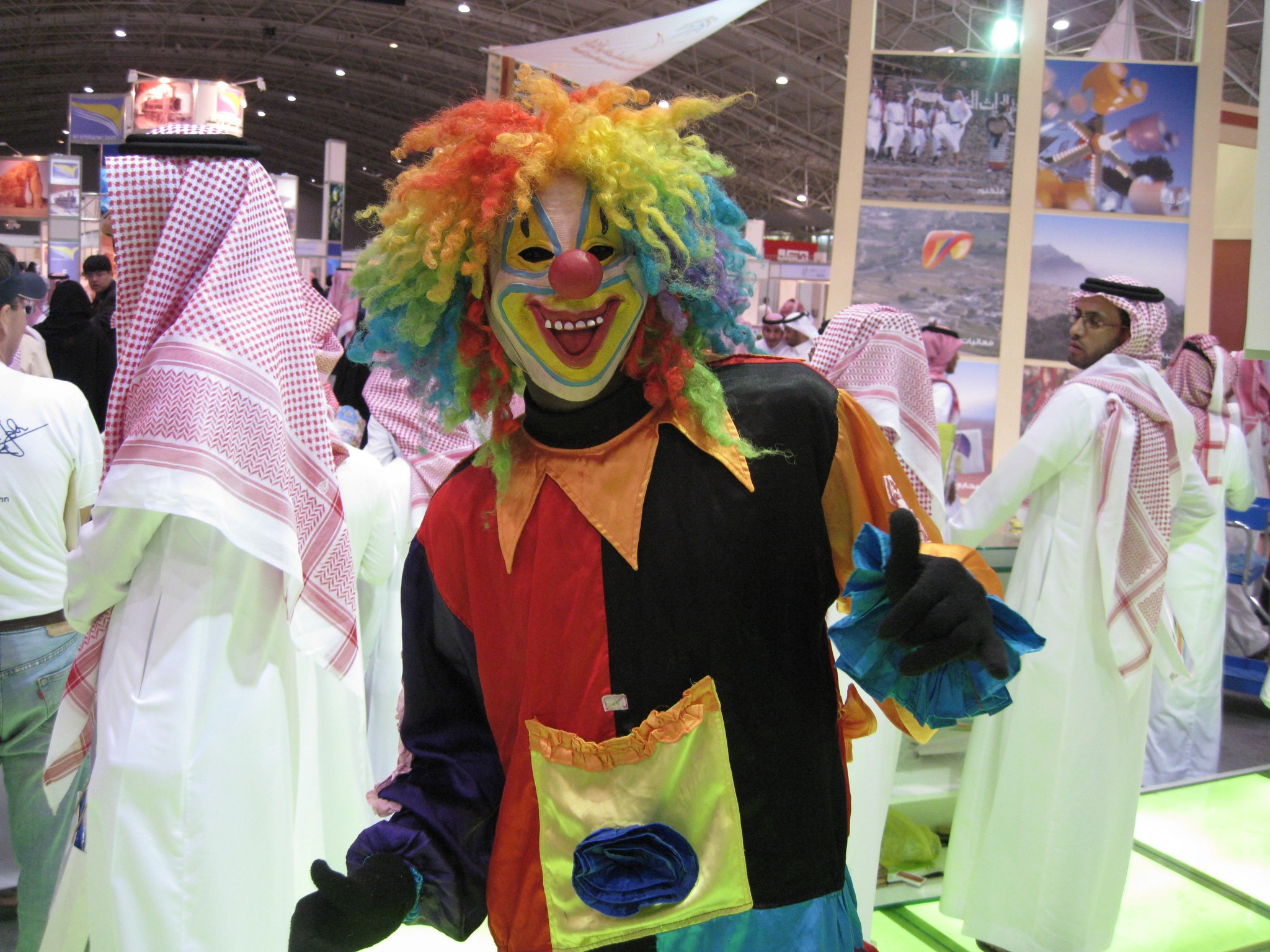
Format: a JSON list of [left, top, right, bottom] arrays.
[[231, 77, 266, 93], [127, 69, 160, 84]]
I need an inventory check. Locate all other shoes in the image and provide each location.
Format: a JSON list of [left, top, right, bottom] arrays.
[[930, 819, 953, 848]]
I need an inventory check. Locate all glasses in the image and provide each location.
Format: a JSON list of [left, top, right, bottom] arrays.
[[10, 304, 33, 314], [1068, 313, 1130, 329]]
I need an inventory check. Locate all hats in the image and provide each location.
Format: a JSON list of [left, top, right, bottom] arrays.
[[0, 254, 48, 309]]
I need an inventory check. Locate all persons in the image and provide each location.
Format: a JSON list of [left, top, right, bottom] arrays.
[[808, 304, 946, 950], [0, 240, 103, 952], [757, 297, 773, 324], [9, 292, 54, 379], [755, 313, 793, 359], [779, 312, 820, 365], [288, 65, 1044, 952], [942, 274, 1215, 952], [1143, 333, 1260, 787], [32, 280, 107, 436], [920, 324, 961, 517], [36, 272, 70, 325], [41, 123, 365, 952], [83, 255, 117, 387]]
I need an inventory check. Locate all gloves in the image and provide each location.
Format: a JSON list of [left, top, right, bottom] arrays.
[[285, 848, 416, 952], [877, 507, 1009, 679]]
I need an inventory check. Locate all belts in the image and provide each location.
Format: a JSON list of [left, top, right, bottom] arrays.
[[0, 608, 67, 633]]
